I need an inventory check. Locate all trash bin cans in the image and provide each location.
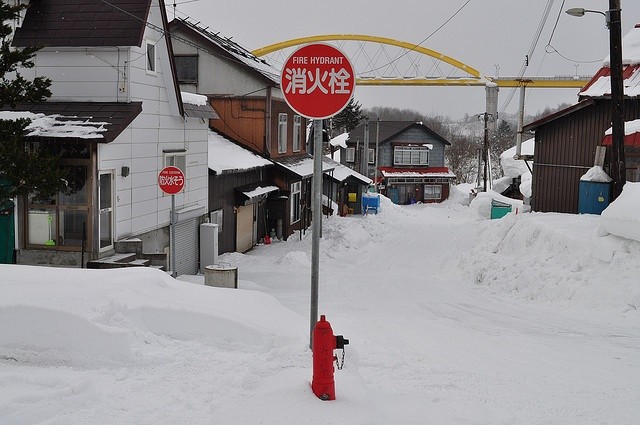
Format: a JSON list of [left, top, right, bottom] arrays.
[[578, 179, 610, 214]]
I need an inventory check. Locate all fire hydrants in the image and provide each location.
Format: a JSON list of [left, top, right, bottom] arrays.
[[311, 314, 349, 402]]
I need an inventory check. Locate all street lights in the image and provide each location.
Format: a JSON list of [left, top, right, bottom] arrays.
[[565, 6, 626, 203]]
